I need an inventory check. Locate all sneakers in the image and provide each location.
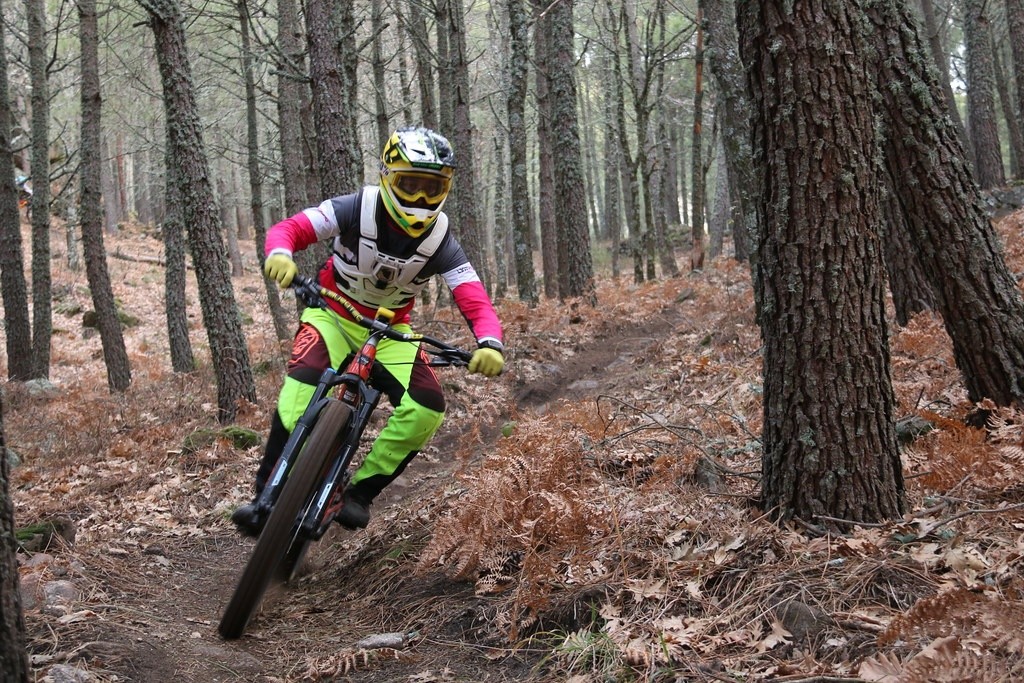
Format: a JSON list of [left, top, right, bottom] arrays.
[[233, 501, 258, 526], [336, 493, 370, 531]]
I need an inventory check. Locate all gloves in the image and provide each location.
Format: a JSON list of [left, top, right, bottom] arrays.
[[468, 336, 504, 378], [263, 253, 298, 288]]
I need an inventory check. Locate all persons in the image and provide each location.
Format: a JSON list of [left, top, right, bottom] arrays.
[[230, 127, 506, 543]]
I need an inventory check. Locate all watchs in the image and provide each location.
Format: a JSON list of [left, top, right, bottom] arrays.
[[478, 340, 504, 356]]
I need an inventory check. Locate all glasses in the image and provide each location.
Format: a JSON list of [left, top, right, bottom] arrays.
[[380, 159, 449, 206]]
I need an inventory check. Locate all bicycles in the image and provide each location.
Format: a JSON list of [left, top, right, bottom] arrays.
[[219, 261, 503, 641]]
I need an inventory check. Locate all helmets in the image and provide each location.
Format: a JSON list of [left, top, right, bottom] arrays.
[[381, 125, 457, 238]]
